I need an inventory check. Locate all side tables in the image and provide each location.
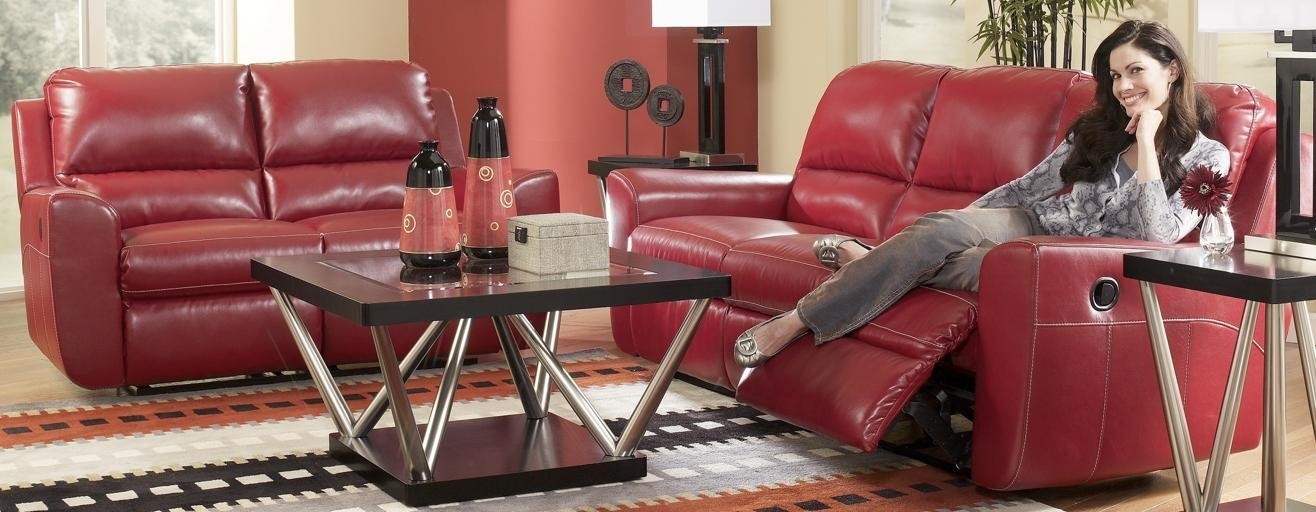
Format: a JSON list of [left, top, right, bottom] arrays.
[[1125, 245, 1313, 512], [589, 158, 759, 208]]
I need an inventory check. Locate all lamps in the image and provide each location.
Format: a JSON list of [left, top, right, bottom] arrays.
[[650, 1, 769, 165]]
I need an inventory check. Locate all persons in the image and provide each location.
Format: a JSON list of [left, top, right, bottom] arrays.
[[732, 20, 1231, 368]]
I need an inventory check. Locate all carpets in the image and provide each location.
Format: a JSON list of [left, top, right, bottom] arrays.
[[0, 346, 1067, 512]]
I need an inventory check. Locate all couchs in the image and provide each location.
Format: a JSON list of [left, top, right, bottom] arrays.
[[610, 59, 1267, 493], [11, 59, 562, 395]]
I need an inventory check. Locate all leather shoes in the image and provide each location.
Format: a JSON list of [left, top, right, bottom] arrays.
[[733, 310, 810, 367], [813, 232, 879, 271]]
